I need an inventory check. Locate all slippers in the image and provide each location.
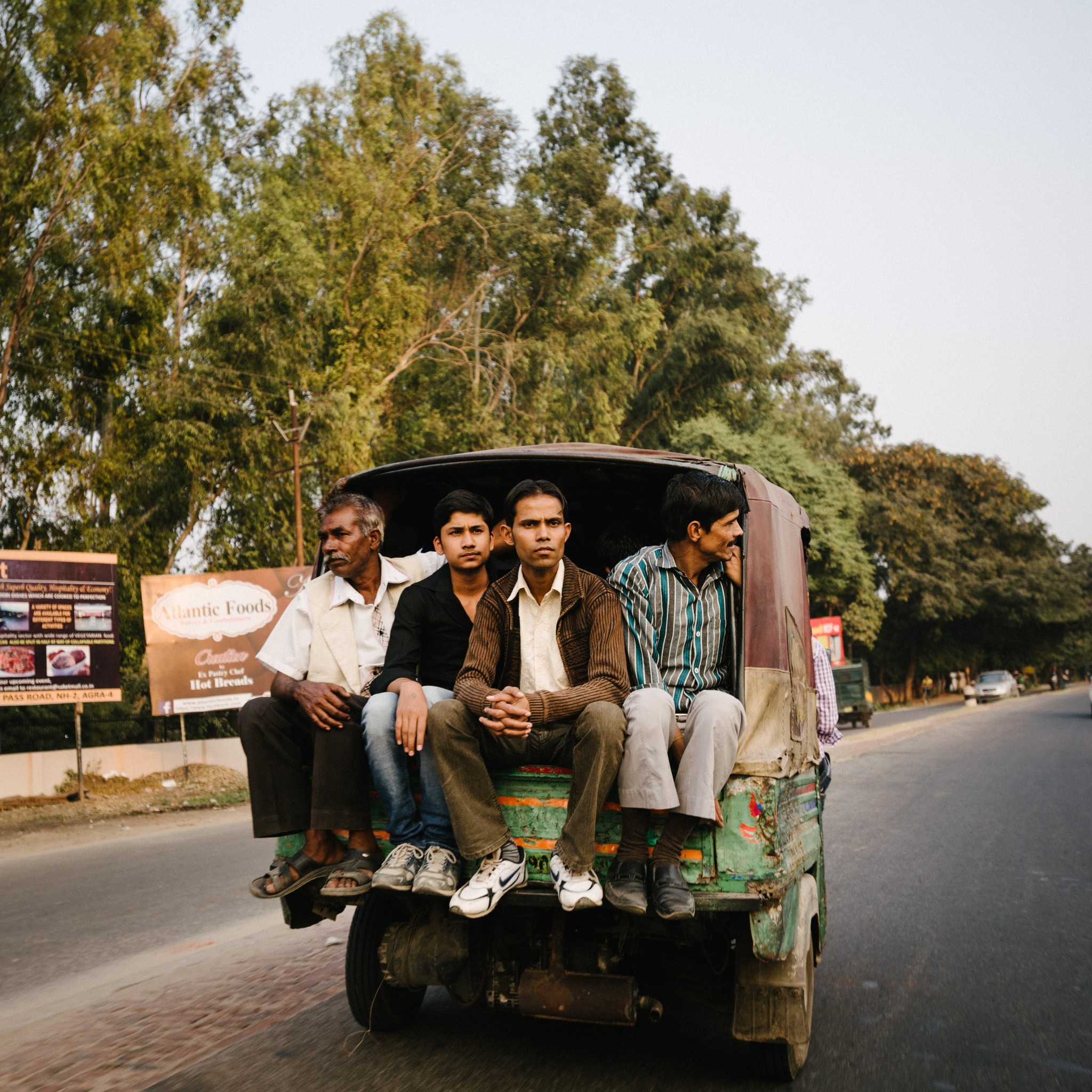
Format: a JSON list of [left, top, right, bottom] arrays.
[[321, 849, 385, 896], [250, 850, 340, 898]]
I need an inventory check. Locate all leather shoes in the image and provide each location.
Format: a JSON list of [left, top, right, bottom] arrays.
[[606, 856, 647, 915], [649, 862, 696, 919]]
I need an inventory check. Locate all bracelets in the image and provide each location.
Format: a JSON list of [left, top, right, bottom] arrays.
[[673, 736, 683, 742]]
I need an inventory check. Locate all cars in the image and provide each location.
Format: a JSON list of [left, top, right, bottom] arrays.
[[968, 669, 1020, 704]]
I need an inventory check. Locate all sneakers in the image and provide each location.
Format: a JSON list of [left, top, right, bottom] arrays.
[[548, 850, 604, 911], [371, 843, 422, 892], [412, 844, 462, 896], [449, 847, 526, 918]]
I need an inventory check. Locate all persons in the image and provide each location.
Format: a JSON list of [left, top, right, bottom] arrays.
[[922, 676, 933, 696], [362, 490, 514, 895], [237, 492, 507, 898], [1086, 669, 1091, 683], [426, 479, 631, 918], [606, 470, 744, 919], [958, 671, 965, 693], [1049, 665, 1061, 691], [598, 521, 665, 574]]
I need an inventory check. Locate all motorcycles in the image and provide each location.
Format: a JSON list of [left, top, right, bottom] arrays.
[[303, 442, 841, 1083], [831, 656, 874, 728]]
